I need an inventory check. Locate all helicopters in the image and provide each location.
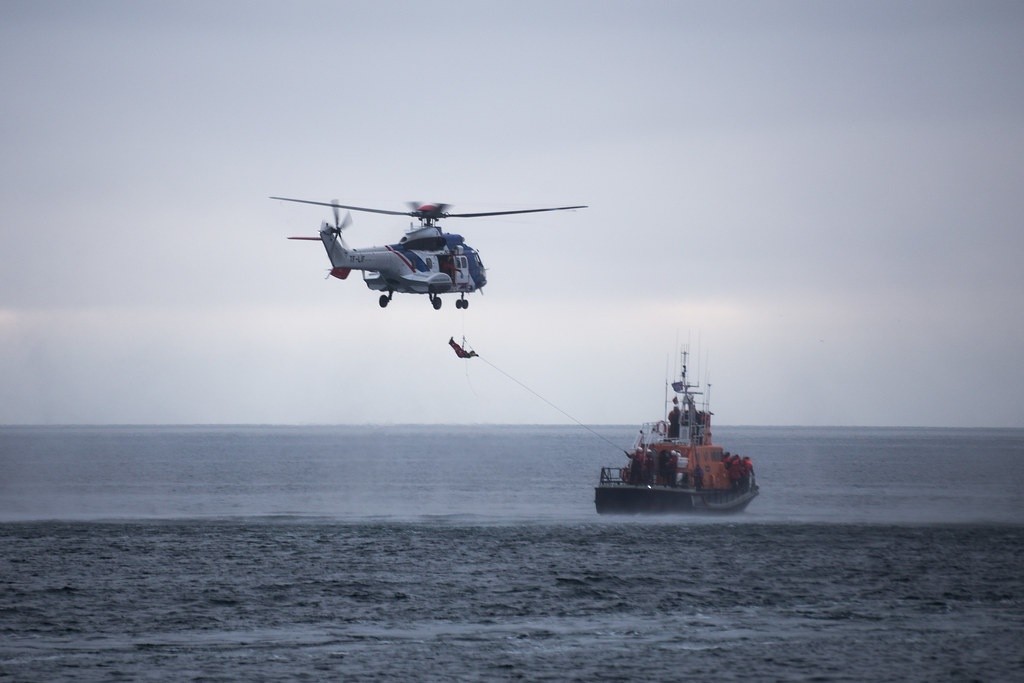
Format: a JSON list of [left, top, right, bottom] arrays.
[[272, 197, 588, 309]]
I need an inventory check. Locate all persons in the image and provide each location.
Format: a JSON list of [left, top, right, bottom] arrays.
[[724, 451, 755, 490], [692, 465, 704, 490], [450, 337, 479, 358], [623, 446, 677, 488]]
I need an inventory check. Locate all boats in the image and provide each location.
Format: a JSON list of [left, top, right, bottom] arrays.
[[592, 346, 760, 513]]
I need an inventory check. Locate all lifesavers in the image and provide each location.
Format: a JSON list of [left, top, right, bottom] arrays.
[[657, 420, 669, 436]]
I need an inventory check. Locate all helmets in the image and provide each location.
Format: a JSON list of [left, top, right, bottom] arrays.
[[671, 450, 681, 457], [637, 447, 643, 451], [646, 449, 652, 453]]
